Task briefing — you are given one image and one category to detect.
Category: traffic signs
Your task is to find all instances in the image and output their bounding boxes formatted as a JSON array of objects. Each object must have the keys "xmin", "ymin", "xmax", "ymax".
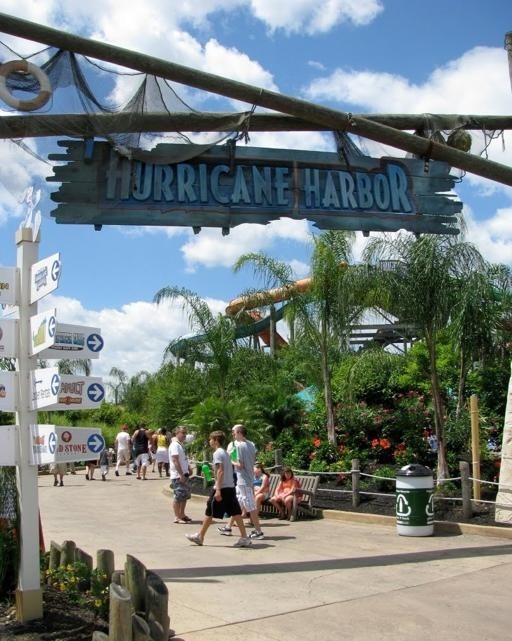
[
  {"xmin": 33, "ymin": 375, "xmax": 105, "ymax": 412},
  {"xmin": 29, "ymin": 308, "xmax": 58, "ymax": 357},
  {"xmin": 1, "ymin": 372, "xmax": 17, "ymax": 412},
  {"xmin": 1, "ymin": 320, "xmax": 18, "ymax": 357},
  {"xmin": 0, "ymin": 266, "xmax": 21, "ymax": 302},
  {"xmin": 30, "ymin": 423, "xmax": 56, "ymax": 464},
  {"xmin": 54, "ymin": 427, "xmax": 106, "ymax": 463},
  {"xmin": 27, "ymin": 367, "xmax": 59, "ymax": 415},
  {"xmin": 39, "ymin": 324, "xmax": 104, "ymax": 360},
  {"xmin": 30, "ymin": 254, "xmax": 63, "ymax": 304}
]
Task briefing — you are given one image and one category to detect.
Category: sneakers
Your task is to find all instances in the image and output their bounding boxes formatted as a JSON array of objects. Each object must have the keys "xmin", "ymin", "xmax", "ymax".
[
  {"xmin": 235, "ymin": 537, "xmax": 252, "ymax": 547},
  {"xmin": 185, "ymin": 533, "xmax": 203, "ymax": 545},
  {"xmin": 218, "ymin": 526, "xmax": 232, "ymax": 535},
  {"xmin": 249, "ymin": 531, "xmax": 265, "ymax": 539},
  {"xmin": 182, "ymin": 516, "xmax": 192, "ymax": 521}
]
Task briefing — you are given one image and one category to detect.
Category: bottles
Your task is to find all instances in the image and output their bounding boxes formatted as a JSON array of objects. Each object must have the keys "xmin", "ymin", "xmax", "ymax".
[
  {"xmin": 230, "ymin": 448, "xmax": 238, "ymax": 462},
  {"xmin": 201, "ymin": 463, "xmax": 212, "ymax": 483}
]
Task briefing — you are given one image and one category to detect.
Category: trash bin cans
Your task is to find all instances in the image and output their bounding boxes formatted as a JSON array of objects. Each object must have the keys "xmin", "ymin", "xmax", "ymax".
[{"xmin": 395, "ymin": 464, "xmax": 435, "ymax": 537}]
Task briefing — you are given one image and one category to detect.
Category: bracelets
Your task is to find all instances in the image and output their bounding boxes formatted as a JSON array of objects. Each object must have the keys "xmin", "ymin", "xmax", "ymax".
[{"xmin": 216, "ymin": 488, "xmax": 221, "ymax": 490}]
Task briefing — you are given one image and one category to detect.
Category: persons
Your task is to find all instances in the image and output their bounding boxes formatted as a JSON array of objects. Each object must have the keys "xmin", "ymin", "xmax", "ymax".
[
  {"xmin": 217, "ymin": 426, "xmax": 264, "ymax": 540},
  {"xmin": 269, "ymin": 468, "xmax": 304, "ymax": 520},
  {"xmin": 49, "ymin": 464, "xmax": 65, "ymax": 486},
  {"xmin": 184, "ymin": 431, "xmax": 252, "ymax": 547},
  {"xmin": 63, "ymin": 463, "xmax": 77, "ymax": 475},
  {"xmin": 201, "ymin": 424, "xmax": 257, "ymax": 527},
  {"xmin": 232, "ymin": 463, "xmax": 270, "ymax": 528},
  {"xmin": 85, "ymin": 422, "xmax": 195, "ymax": 524}
]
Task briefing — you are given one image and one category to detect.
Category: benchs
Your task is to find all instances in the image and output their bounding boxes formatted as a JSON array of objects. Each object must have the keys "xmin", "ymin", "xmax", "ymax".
[{"xmin": 260, "ymin": 473, "xmax": 323, "ymax": 522}]
